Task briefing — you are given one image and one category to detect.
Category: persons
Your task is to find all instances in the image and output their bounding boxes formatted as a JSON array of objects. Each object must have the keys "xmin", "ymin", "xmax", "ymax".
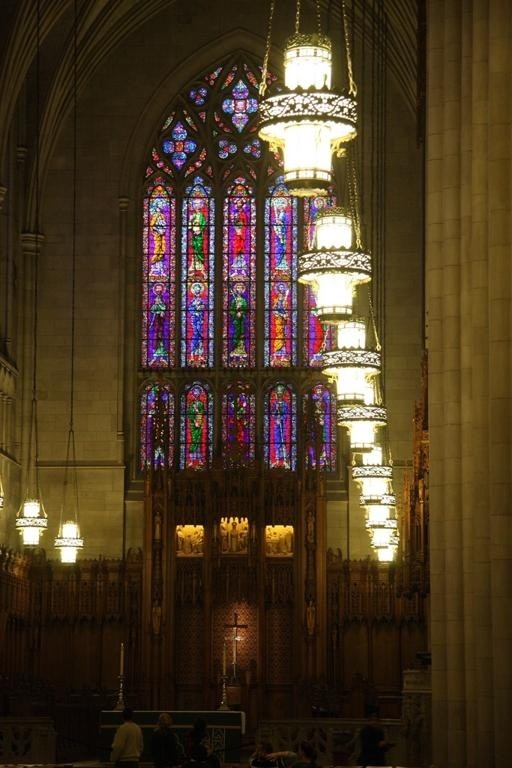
[{"xmin": 109, "ymin": 706, "xmax": 397, "ymax": 767}]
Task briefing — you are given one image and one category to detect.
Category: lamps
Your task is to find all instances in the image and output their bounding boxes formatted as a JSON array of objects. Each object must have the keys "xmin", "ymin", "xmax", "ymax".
[
  {"xmin": 258, "ymin": 1, "xmax": 360, "ymax": 202},
  {"xmin": 309, "ymin": 283, "xmax": 404, "ymax": 570},
  {"xmin": 297, "ymin": 140, "xmax": 372, "ymax": 319},
  {"xmin": 16, "ymin": 0, "xmax": 84, "ymax": 566}
]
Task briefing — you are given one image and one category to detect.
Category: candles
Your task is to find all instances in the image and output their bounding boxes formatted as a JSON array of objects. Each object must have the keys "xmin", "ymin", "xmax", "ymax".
[
  {"xmin": 222, "ymin": 643, "xmax": 227, "ymax": 675},
  {"xmin": 119, "ymin": 643, "xmax": 125, "ymax": 676}
]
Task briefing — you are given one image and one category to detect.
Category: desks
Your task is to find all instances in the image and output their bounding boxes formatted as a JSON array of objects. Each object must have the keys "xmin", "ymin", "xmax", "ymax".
[{"xmin": 99, "ymin": 710, "xmax": 247, "ymax": 760}]
